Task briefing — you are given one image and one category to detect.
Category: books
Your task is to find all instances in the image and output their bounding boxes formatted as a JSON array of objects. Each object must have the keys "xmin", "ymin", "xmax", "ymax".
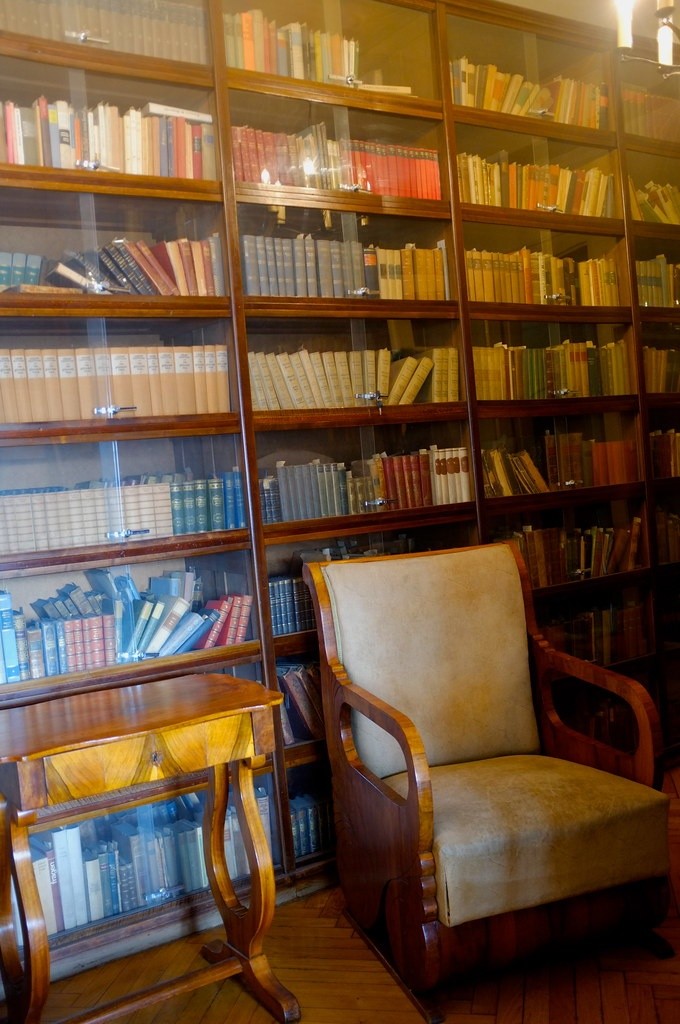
[{"xmin": 0, "ymin": 1, "xmax": 680, "ymax": 945}]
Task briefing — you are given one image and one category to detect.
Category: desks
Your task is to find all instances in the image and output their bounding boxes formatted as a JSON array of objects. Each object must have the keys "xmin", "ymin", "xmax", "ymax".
[{"xmin": 0, "ymin": 672, "xmax": 302, "ymax": 1024}]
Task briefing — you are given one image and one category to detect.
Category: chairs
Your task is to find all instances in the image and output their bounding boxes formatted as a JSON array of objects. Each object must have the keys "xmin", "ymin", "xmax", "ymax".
[{"xmin": 303, "ymin": 539, "xmax": 679, "ymax": 1024}]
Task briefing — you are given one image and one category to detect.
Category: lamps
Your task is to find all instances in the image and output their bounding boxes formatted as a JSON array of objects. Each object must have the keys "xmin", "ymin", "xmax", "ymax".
[{"xmin": 615, "ymin": 0, "xmax": 680, "ymax": 80}]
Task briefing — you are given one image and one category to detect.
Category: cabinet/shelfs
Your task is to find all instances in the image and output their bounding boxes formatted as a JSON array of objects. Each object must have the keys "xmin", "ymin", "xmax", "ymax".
[{"xmin": 0, "ymin": 2, "xmax": 680, "ymax": 1000}]
